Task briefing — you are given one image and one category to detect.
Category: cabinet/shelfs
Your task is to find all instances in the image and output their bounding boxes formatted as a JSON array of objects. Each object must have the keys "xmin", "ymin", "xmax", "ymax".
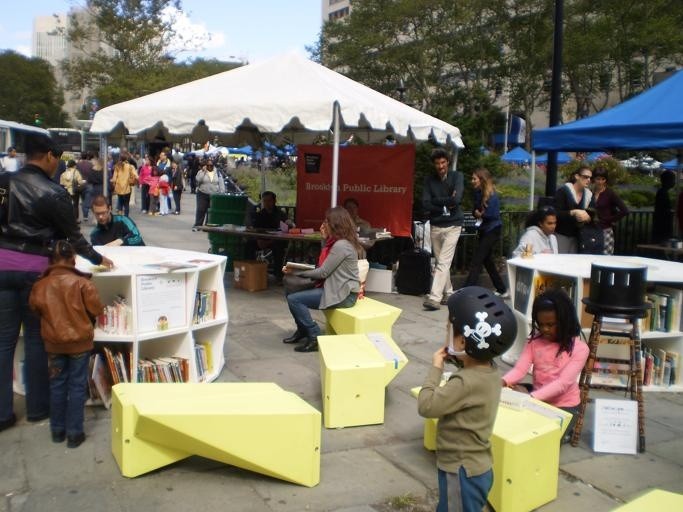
[
  {"xmin": 11, "ymin": 244, "xmax": 232, "ymax": 407},
  {"xmin": 498, "ymin": 253, "xmax": 682, "ymax": 394}
]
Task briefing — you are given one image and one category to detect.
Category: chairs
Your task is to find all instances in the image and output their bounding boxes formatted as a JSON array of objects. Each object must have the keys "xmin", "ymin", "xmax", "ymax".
[{"xmin": 568, "ymin": 261, "xmax": 654, "ymax": 454}]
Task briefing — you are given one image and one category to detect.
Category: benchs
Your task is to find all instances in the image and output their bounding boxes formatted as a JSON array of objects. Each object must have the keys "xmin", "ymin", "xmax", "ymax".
[
  {"xmin": 318, "ymin": 294, "xmax": 403, "ymax": 337},
  {"xmin": 610, "ymin": 486, "xmax": 682, "ymax": 512},
  {"xmin": 109, "ymin": 381, "xmax": 322, "ymax": 490},
  {"xmin": 408, "ymin": 373, "xmax": 574, "ymax": 512},
  {"xmin": 315, "ymin": 332, "xmax": 409, "ymax": 430}
]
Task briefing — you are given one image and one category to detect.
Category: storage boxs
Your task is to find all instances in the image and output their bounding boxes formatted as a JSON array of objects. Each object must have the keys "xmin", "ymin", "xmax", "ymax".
[
  {"xmin": 362, "ymin": 263, "xmax": 393, "ymax": 295},
  {"xmin": 233, "ymin": 258, "xmax": 268, "ymax": 293}
]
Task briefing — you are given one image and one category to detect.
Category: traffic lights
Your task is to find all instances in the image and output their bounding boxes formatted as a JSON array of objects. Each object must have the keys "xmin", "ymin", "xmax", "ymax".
[
  {"xmin": 34, "ymin": 113, "xmax": 39, "ymax": 123},
  {"xmin": 81, "ymin": 104, "xmax": 85, "ymax": 113}
]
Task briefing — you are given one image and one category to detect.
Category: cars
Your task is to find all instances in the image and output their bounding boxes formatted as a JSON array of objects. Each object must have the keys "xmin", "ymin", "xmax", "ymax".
[{"xmin": 180, "ymin": 160, "xmax": 189, "ymax": 178}]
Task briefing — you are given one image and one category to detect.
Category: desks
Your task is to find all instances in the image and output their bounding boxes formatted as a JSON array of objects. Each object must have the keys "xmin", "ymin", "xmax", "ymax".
[{"xmin": 189, "ymin": 223, "xmax": 393, "ymax": 284}]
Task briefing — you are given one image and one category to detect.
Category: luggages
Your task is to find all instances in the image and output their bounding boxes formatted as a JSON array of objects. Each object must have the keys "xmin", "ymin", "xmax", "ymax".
[{"xmin": 392, "ymin": 221, "xmax": 433, "ymax": 297}]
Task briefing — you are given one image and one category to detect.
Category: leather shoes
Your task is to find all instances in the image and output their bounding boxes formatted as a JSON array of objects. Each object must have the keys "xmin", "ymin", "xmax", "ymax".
[
  {"xmin": 283, "ymin": 331, "xmax": 305, "ymax": 344},
  {"xmin": 294, "ymin": 339, "xmax": 319, "ymax": 352}
]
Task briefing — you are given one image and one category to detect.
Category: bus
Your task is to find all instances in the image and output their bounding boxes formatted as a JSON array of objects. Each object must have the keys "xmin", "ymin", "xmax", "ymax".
[{"xmin": 0, "ymin": 119, "xmax": 173, "ymax": 175}]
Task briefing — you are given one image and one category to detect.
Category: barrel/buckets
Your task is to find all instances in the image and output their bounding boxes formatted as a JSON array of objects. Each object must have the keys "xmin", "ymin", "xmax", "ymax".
[{"xmin": 207, "ymin": 192, "xmax": 251, "ymax": 272}]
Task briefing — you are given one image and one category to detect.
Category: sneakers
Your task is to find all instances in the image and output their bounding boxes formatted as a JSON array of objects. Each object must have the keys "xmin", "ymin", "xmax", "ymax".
[
  {"xmin": 67, "ymin": 431, "xmax": 86, "ymax": 449},
  {"xmin": 26, "ymin": 412, "xmax": 51, "ymax": 423},
  {"xmin": 423, "ymin": 298, "xmax": 441, "ymax": 311},
  {"xmin": 440, "ymin": 294, "xmax": 451, "ymax": 305},
  {"xmin": 140, "ymin": 207, "xmax": 180, "ymax": 217},
  {"xmin": 275, "ymin": 278, "xmax": 283, "ymax": 287},
  {"xmin": 493, "ymin": 291, "xmax": 509, "ymax": 299},
  {"xmin": 0, "ymin": 412, "xmax": 17, "ymax": 433},
  {"xmin": 192, "ymin": 227, "xmax": 201, "ymax": 232},
  {"xmin": 50, "ymin": 428, "xmax": 67, "ymax": 444}
]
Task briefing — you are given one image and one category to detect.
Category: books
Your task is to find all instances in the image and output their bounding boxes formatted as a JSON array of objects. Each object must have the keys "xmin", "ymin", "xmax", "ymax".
[
  {"xmin": 284, "ymin": 262, "xmax": 316, "ymax": 271},
  {"xmin": 88, "ymin": 288, "xmax": 216, "ymax": 410},
  {"xmin": 594, "ymin": 285, "xmax": 683, "ymax": 386}
]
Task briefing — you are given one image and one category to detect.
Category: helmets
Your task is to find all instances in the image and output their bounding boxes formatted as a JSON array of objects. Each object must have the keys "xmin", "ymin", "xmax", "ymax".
[{"xmin": 446, "ymin": 284, "xmax": 519, "ymax": 361}]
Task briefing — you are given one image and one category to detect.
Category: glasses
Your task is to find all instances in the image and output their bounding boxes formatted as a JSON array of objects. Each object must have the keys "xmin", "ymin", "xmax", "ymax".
[
  {"xmin": 580, "ymin": 174, "xmax": 593, "ymax": 181},
  {"xmin": 541, "ymin": 204, "xmax": 554, "ymax": 211}
]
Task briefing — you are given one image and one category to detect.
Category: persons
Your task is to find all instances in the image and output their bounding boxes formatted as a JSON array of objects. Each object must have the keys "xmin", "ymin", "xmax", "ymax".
[
  {"xmin": 283, "ymin": 208, "xmax": 363, "ymax": 353},
  {"xmin": 462, "ymin": 168, "xmax": 512, "ymax": 300},
  {"xmin": 422, "ymin": 149, "xmax": 465, "ymax": 309},
  {"xmin": 0, "ymin": 134, "xmax": 114, "ymax": 431},
  {"xmin": 502, "ymin": 286, "xmax": 592, "ymax": 431},
  {"xmin": 651, "ymin": 170, "xmax": 675, "ymax": 244},
  {"xmin": 0, "ymin": 144, "xmax": 245, "ymax": 245},
  {"xmin": 417, "ymin": 286, "xmax": 517, "ymax": 512},
  {"xmin": 511, "ymin": 206, "xmax": 558, "ymax": 253},
  {"xmin": 245, "ymin": 190, "xmax": 288, "ymax": 268},
  {"xmin": 29, "ymin": 238, "xmax": 104, "ymax": 448},
  {"xmin": 343, "ymin": 196, "xmax": 370, "ymax": 230},
  {"xmin": 592, "ymin": 166, "xmax": 627, "ymax": 254},
  {"xmin": 555, "ymin": 167, "xmax": 595, "ymax": 252}
]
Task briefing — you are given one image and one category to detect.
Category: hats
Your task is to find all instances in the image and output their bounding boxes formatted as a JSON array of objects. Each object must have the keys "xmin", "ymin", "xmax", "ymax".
[{"xmin": 590, "ymin": 166, "xmax": 610, "ymax": 182}]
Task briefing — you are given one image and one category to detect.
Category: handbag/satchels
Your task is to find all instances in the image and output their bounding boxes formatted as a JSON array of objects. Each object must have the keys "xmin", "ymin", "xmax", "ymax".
[
  {"xmin": 575, "ymin": 215, "xmax": 605, "ymax": 255},
  {"xmin": 71, "ymin": 168, "xmax": 89, "ymax": 195},
  {"xmin": 128, "ymin": 164, "xmax": 136, "ymax": 187}
]
